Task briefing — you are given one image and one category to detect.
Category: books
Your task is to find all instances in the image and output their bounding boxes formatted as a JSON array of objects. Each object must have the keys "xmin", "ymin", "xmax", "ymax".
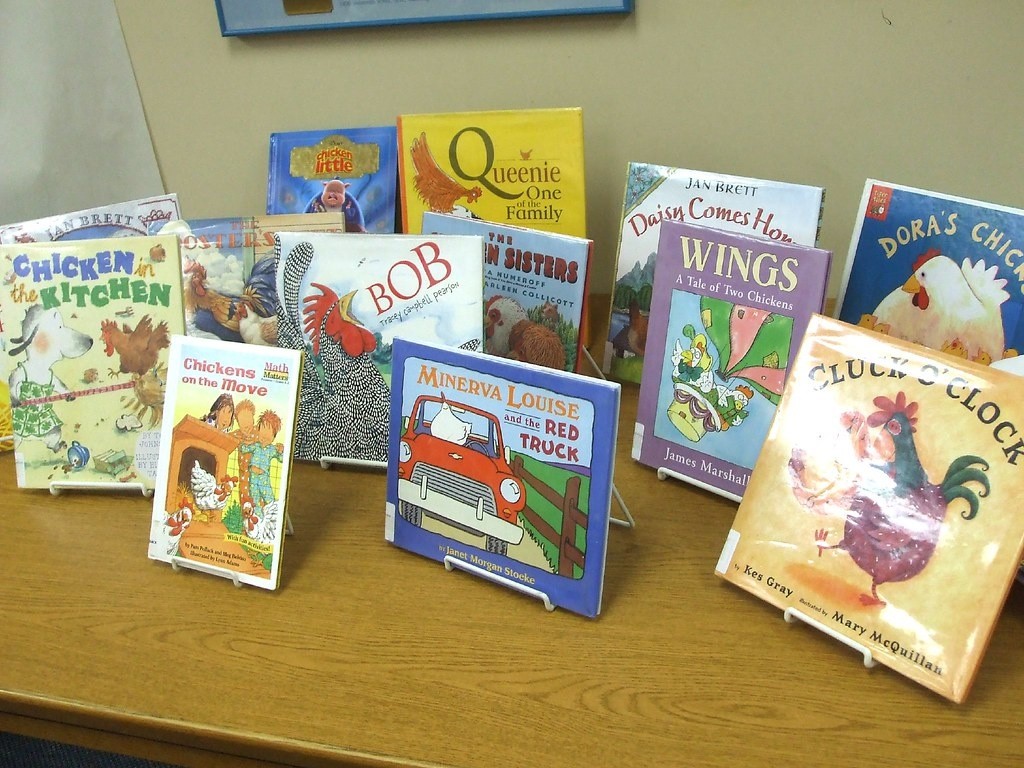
[{"xmin": 1, "ymin": 105, "xmax": 1024, "ymax": 706}]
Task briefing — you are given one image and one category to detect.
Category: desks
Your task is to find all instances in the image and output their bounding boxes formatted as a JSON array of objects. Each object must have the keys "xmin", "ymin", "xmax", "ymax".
[{"xmin": 0, "ymin": 290, "xmax": 1024, "ymax": 768}]
